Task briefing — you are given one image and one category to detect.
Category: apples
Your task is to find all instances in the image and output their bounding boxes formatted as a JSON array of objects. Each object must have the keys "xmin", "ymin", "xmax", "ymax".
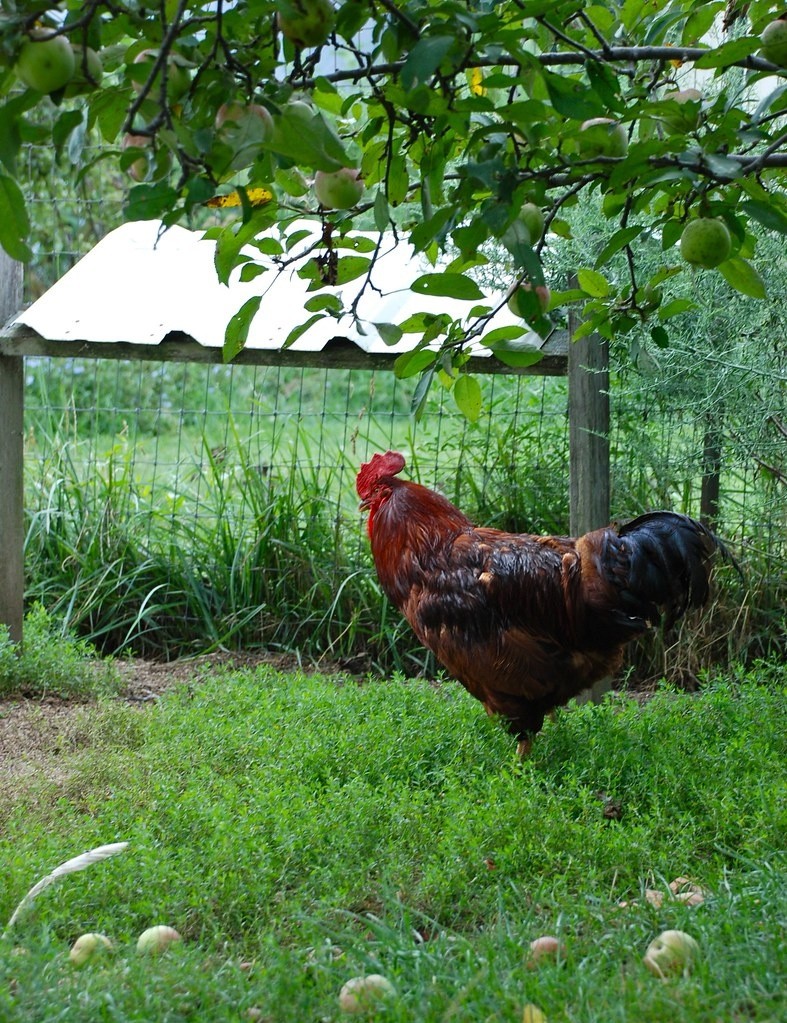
[
  {"xmin": 0, "ymin": 0, "xmax": 787, "ymax": 268},
  {"xmin": 8, "ymin": 875, "xmax": 714, "ymax": 1023}
]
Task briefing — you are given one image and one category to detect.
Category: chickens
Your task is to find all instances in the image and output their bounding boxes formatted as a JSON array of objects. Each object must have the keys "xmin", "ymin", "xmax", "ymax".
[{"xmin": 356, "ymin": 450, "xmax": 744, "ymax": 760}]
